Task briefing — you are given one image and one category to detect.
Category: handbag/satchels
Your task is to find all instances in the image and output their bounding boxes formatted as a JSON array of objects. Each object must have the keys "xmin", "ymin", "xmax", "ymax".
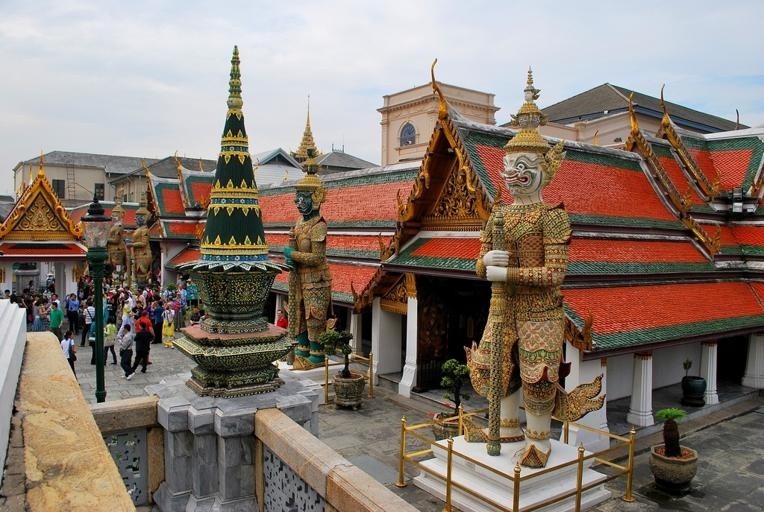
[{"xmin": 69, "ymin": 350, "xmax": 77, "ymax": 362}]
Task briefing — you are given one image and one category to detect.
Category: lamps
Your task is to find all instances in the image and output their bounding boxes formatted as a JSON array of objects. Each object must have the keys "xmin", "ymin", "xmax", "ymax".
[
  {"xmin": 730, "ymin": 185, "xmax": 745, "ymax": 201},
  {"xmin": 733, "ymin": 200, "xmax": 744, "ymax": 214}
]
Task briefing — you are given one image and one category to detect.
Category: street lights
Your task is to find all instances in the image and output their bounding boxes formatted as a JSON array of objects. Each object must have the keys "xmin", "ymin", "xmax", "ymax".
[
  {"xmin": 81, "ymin": 190, "xmax": 116, "ymax": 403},
  {"xmin": 115, "ymin": 263, "xmax": 123, "ymax": 282}
]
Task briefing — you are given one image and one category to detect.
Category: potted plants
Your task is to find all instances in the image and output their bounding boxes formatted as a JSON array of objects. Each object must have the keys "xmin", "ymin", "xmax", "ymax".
[
  {"xmin": 318, "ymin": 328, "xmax": 365, "ymax": 412},
  {"xmin": 679, "ymin": 356, "xmax": 707, "ymax": 407},
  {"xmin": 647, "ymin": 403, "xmax": 700, "ymax": 499},
  {"xmin": 431, "ymin": 355, "xmax": 470, "ymax": 440}
]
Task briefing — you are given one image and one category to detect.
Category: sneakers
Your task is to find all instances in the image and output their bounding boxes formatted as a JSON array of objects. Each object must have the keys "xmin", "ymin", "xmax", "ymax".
[{"xmin": 121, "ymin": 369, "xmax": 146, "ymax": 380}]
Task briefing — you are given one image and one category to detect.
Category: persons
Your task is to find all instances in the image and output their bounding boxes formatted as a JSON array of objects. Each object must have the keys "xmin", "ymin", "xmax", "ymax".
[
  {"xmin": 3, "ymin": 265, "xmax": 199, "ymax": 382},
  {"xmin": 285, "ymin": 148, "xmax": 333, "ymax": 373},
  {"xmin": 106, "ymin": 193, "xmax": 128, "ymax": 274},
  {"xmin": 276, "ymin": 310, "xmax": 288, "ymax": 363},
  {"xmin": 461, "ymin": 64, "xmax": 606, "ymax": 468},
  {"xmin": 125, "ymin": 190, "xmax": 154, "ymax": 281}
]
